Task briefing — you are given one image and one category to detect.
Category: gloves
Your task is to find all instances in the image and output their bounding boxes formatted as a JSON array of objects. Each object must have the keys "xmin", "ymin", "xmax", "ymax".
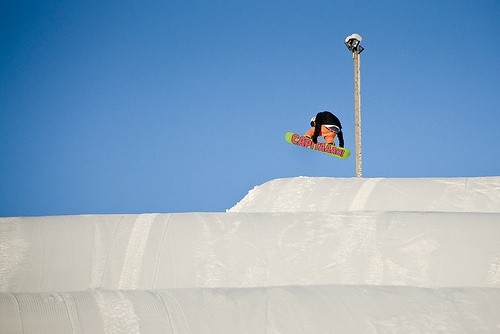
[
  {"xmin": 313, "ymin": 135, "xmax": 317, "ymax": 145},
  {"xmin": 339, "ymin": 141, "xmax": 345, "ymax": 147}
]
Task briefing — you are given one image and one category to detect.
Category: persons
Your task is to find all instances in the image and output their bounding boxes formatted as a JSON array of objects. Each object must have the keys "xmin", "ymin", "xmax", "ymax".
[{"xmin": 303, "ymin": 111, "xmax": 344, "ymax": 148}]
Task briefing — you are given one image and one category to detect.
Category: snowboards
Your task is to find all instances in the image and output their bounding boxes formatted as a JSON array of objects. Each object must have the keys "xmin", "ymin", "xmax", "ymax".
[{"xmin": 285, "ymin": 131, "xmax": 351, "ymax": 160}]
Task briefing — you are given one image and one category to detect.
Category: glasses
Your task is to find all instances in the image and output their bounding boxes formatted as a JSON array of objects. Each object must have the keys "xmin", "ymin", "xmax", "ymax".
[{"xmin": 310, "ymin": 121, "xmax": 314, "ymax": 126}]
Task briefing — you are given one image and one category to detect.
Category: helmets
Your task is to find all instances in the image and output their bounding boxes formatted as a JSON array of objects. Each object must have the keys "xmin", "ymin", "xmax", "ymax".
[{"xmin": 309, "ymin": 116, "xmax": 315, "ymax": 126}]
